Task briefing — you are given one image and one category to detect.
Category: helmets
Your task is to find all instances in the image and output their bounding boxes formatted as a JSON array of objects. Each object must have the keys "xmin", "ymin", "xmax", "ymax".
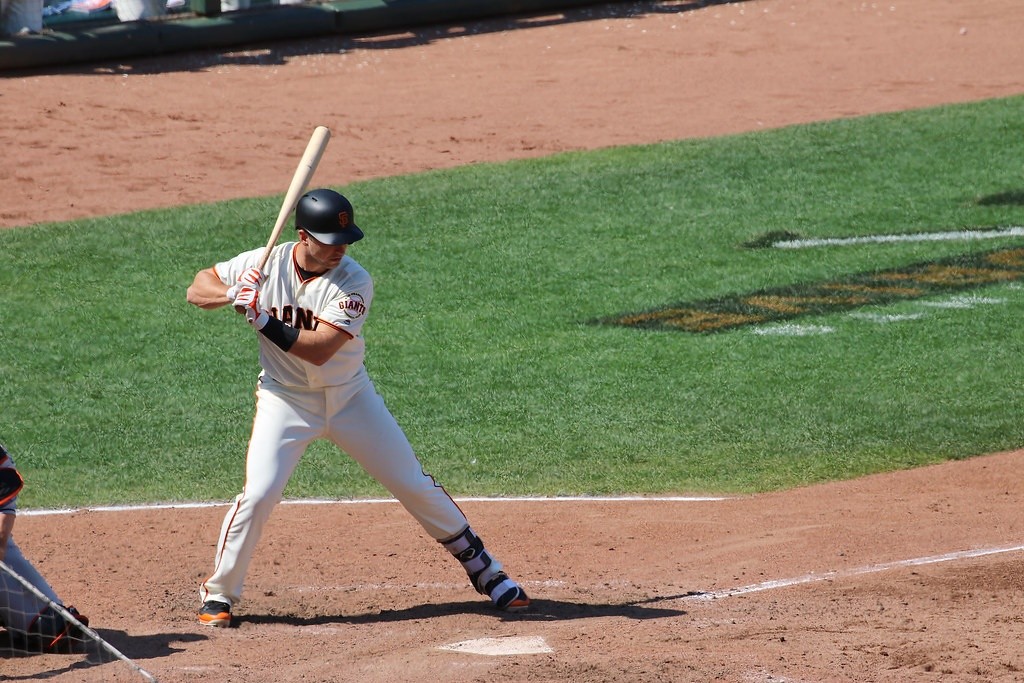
[{"xmin": 295, "ymin": 189, "xmax": 364, "ymax": 246}]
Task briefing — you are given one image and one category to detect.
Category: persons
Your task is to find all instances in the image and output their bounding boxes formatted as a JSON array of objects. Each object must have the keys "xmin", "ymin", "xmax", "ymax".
[
  {"xmin": 0, "ymin": 443, "xmax": 89, "ymax": 653},
  {"xmin": 186, "ymin": 190, "xmax": 531, "ymax": 628}
]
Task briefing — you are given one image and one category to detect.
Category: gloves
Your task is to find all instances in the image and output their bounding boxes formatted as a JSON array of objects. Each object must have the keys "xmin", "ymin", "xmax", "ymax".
[
  {"xmin": 226, "ymin": 268, "xmax": 271, "ymax": 303},
  {"xmin": 231, "ymin": 286, "xmax": 268, "ymax": 331}
]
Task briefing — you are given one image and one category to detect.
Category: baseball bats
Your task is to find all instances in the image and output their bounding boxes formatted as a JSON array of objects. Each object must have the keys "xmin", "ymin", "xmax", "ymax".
[{"xmin": 234, "ymin": 124, "xmax": 333, "ymax": 315}]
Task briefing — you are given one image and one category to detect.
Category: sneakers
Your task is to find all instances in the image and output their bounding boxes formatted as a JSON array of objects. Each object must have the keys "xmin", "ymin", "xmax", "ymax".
[
  {"xmin": 495, "ymin": 589, "xmax": 531, "ymax": 610},
  {"xmin": 197, "ymin": 600, "xmax": 232, "ymax": 629}
]
